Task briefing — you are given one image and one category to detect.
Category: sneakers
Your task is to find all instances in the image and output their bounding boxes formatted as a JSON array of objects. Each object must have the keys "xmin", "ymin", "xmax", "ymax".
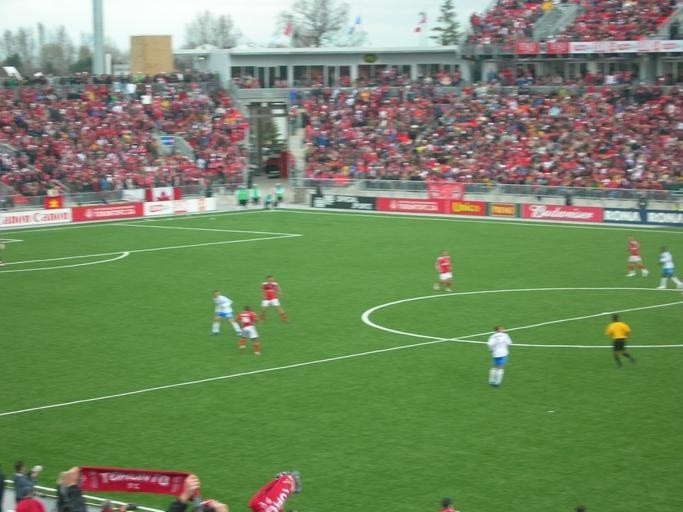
[{"xmin": 433, "ymin": 285, "xmax": 452, "ymax": 291}]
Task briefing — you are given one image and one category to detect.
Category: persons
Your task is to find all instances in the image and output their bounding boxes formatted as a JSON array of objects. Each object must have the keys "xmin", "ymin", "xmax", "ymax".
[
  {"xmin": 623, "ymin": 234, "xmax": 648, "ymax": 278},
  {"xmin": 235, "ymin": 307, "xmax": 261, "ymax": 355},
  {"xmin": 257, "ymin": 274, "xmax": 288, "ymax": 324},
  {"xmin": 487, "ymin": 326, "xmax": 515, "ymax": 388},
  {"xmin": 433, "ymin": 252, "xmax": 454, "ymax": 292},
  {"xmin": 209, "ymin": 290, "xmax": 241, "ymax": 335},
  {"xmin": 603, "ymin": 314, "xmax": 637, "ymax": 368},
  {"xmin": 439, "ymin": 497, "xmax": 460, "ymax": 512},
  {"xmin": 654, "ymin": 245, "xmax": 682, "ymax": 290}
]
